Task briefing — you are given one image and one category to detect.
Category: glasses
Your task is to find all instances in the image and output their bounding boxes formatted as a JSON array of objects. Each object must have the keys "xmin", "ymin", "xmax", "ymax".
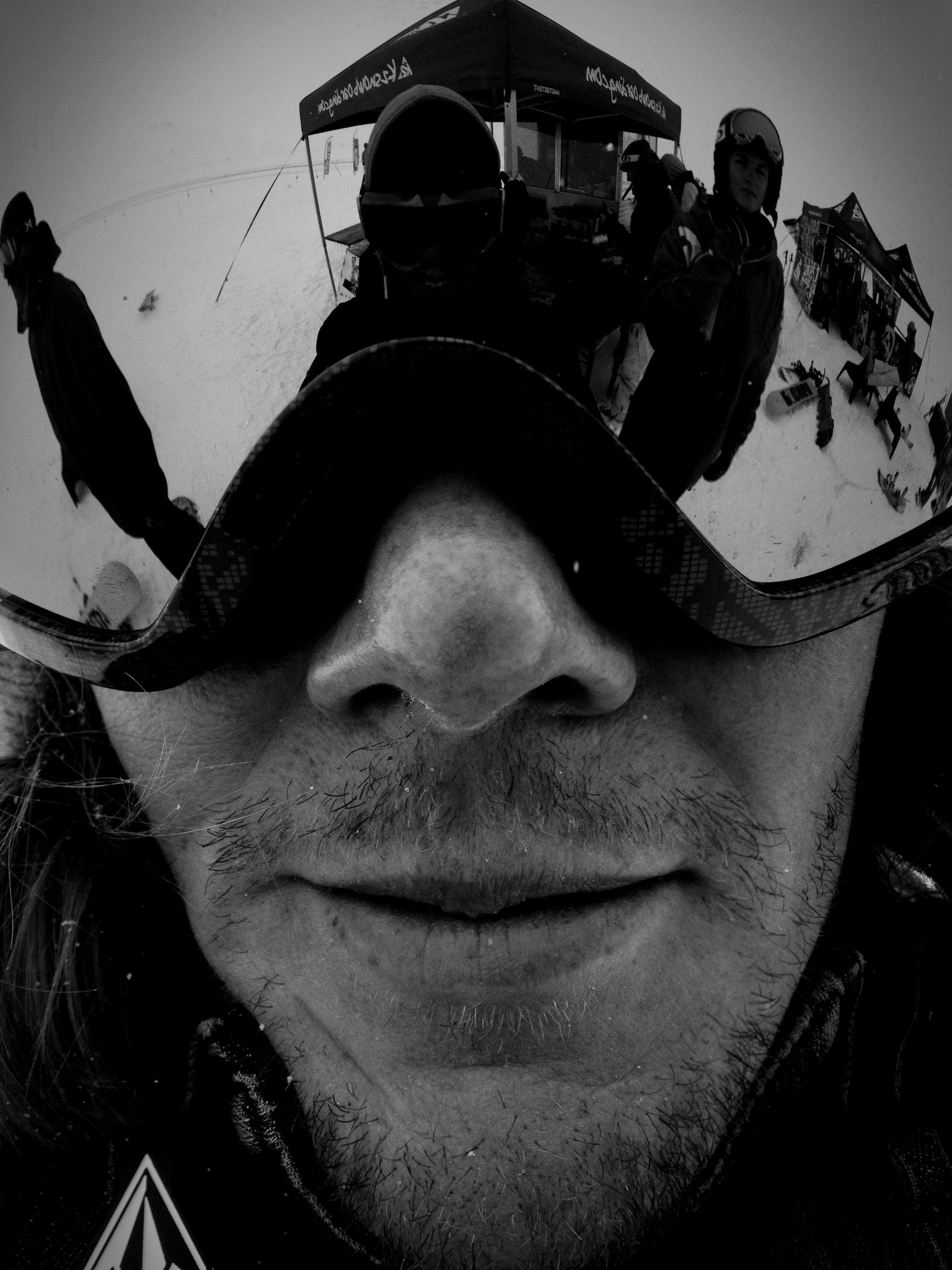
[{"xmin": 1, "ymin": 2, "xmax": 947, "ymax": 688}]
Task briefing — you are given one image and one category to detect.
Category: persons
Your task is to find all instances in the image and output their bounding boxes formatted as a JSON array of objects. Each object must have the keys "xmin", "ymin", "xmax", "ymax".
[
  {"xmin": 293, "ymin": 83, "xmax": 599, "ymax": 433},
  {"xmin": 0, "ymin": 0, "xmax": 952, "ymax": 1268},
  {"xmin": 2, "ymin": 189, "xmax": 208, "ymax": 581},
  {"xmin": 891, "ymin": 322, "xmax": 917, "ymax": 391},
  {"xmin": 600, "ymin": 139, "xmax": 708, "ymax": 424},
  {"xmin": 620, "ymin": 105, "xmax": 783, "ymax": 508}
]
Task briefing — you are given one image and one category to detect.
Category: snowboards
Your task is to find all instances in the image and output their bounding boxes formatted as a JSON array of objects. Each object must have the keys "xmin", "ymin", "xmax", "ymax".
[
  {"xmin": 766, "ymin": 377, "xmax": 818, "ymax": 417},
  {"xmin": 877, "ymin": 468, "xmax": 908, "ymax": 513},
  {"xmin": 79, "ymin": 560, "xmax": 143, "ymax": 629},
  {"xmin": 816, "ymin": 383, "xmax": 834, "ymax": 448}
]
